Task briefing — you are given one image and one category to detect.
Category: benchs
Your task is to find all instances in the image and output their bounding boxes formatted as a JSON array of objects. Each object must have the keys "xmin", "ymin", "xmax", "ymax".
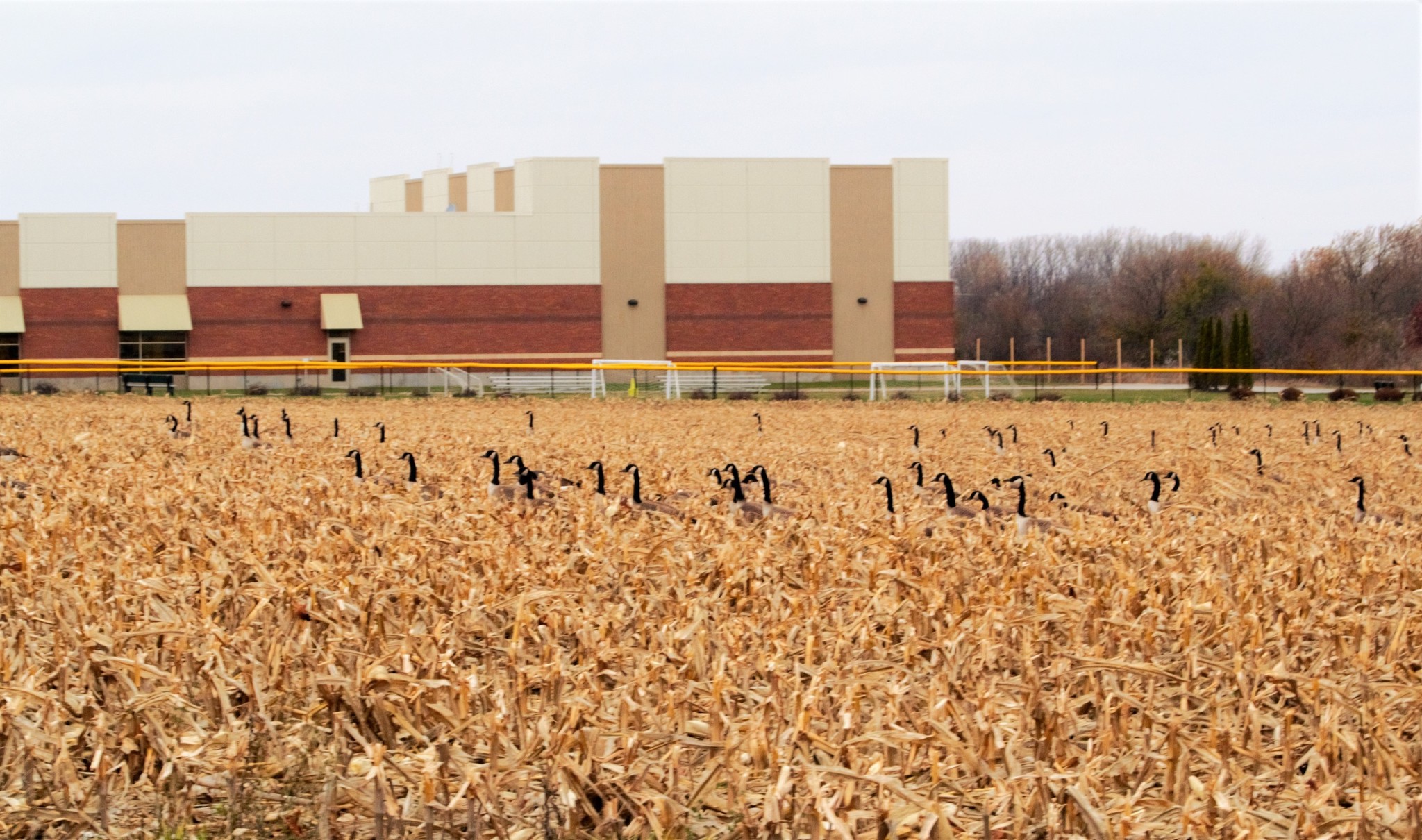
[
  {"xmin": 655, "ymin": 375, "xmax": 771, "ymax": 392},
  {"xmin": 121, "ymin": 373, "xmax": 175, "ymax": 396},
  {"xmin": 486, "ymin": 376, "xmax": 605, "ymax": 394}
]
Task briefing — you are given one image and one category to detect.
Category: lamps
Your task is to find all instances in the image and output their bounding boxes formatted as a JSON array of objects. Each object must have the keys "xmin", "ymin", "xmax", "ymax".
[
  {"xmin": 281, "ymin": 301, "xmax": 292, "ymax": 307},
  {"xmin": 629, "ymin": 298, "xmax": 639, "ymax": 306},
  {"xmin": 857, "ymin": 297, "xmax": 867, "ymax": 304}
]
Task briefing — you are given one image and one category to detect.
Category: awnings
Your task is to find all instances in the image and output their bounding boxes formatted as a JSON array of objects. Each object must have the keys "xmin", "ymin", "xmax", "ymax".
[
  {"xmin": 117, "ymin": 293, "xmax": 193, "ymax": 330},
  {"xmin": 0, "ymin": 296, "xmax": 26, "ymax": 332},
  {"xmin": 320, "ymin": 293, "xmax": 364, "ymax": 329}
]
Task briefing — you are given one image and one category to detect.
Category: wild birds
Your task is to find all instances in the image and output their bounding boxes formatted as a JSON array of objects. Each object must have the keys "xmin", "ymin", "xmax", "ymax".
[{"xmin": 163, "ymin": 398, "xmax": 1413, "ymax": 538}]
